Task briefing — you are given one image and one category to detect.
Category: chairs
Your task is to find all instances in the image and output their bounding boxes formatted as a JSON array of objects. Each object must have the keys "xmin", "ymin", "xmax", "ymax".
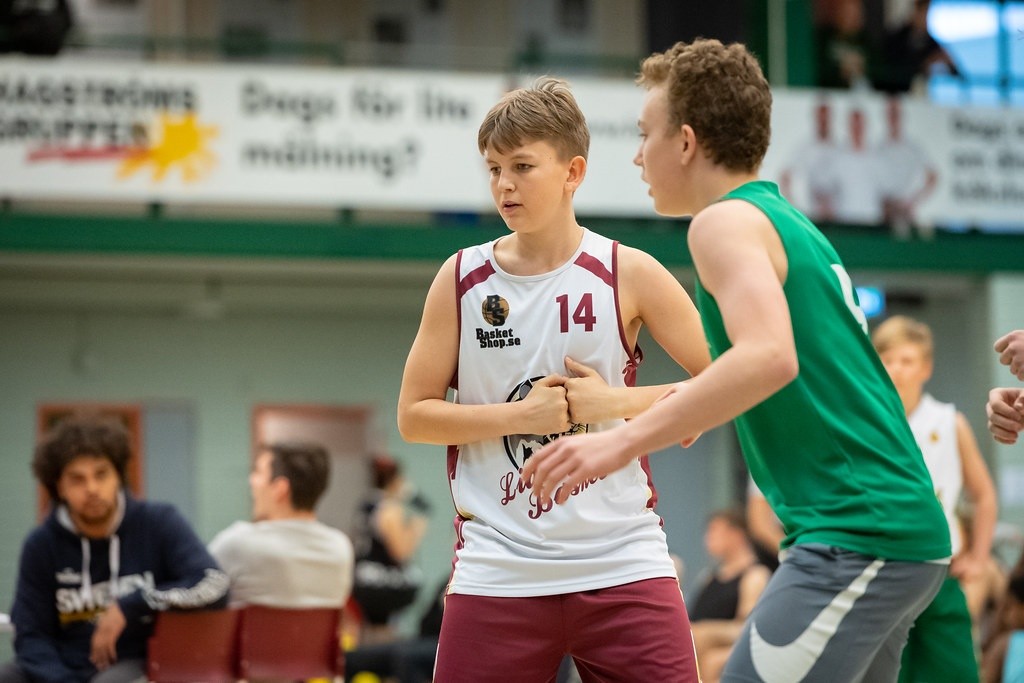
[{"xmin": 144, "ymin": 601, "xmax": 346, "ymax": 683}]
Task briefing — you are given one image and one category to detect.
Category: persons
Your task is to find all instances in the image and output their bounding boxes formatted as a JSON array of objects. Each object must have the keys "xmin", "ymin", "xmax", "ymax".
[
  {"xmin": 683, "ymin": 512, "xmax": 775, "ymax": 683},
  {"xmin": 742, "ymin": 308, "xmax": 1024, "ymax": 683},
  {"xmin": 397, "ymin": 74, "xmax": 717, "ymax": 683},
  {"xmin": 0, "ymin": 0, "xmax": 78, "ymax": 59},
  {"xmin": 520, "ymin": 37, "xmax": 955, "ymax": 683},
  {"xmin": 1, "ymin": 406, "xmax": 232, "ymax": 683},
  {"xmin": 807, "ymin": 0, "xmax": 965, "ymax": 98},
  {"xmin": 205, "ymin": 439, "xmax": 359, "ymax": 611},
  {"xmin": 352, "ymin": 452, "xmax": 430, "ymax": 647}
]
